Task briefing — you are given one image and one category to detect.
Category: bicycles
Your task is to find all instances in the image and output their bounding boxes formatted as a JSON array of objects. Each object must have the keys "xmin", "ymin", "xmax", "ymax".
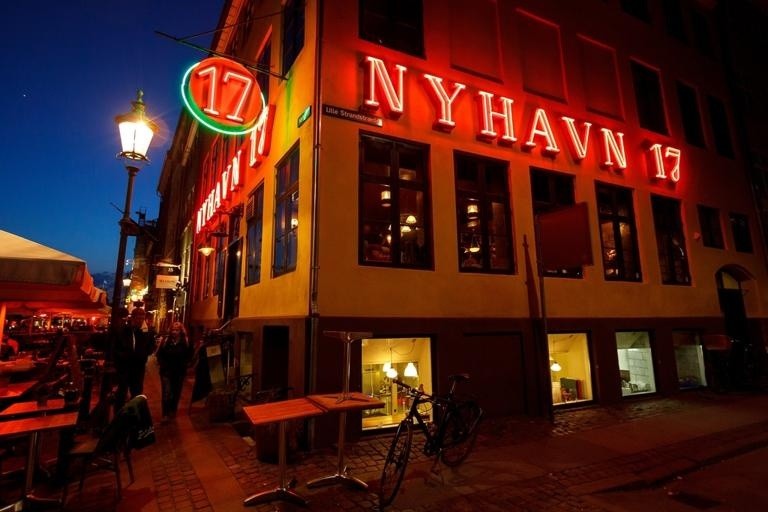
[{"xmin": 379, "ymin": 378, "xmax": 482, "ymax": 505}]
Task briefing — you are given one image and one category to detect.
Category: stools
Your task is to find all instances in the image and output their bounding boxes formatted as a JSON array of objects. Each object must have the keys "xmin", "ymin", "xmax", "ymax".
[{"xmin": 324, "ymin": 329, "xmax": 374, "ymax": 404}]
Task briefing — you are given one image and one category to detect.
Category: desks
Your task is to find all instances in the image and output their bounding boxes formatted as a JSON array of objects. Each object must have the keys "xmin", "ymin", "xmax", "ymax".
[
  {"xmin": 0, "ymin": 378, "xmax": 82, "ymax": 511},
  {"xmin": 243, "ymin": 390, "xmax": 385, "ymax": 507}
]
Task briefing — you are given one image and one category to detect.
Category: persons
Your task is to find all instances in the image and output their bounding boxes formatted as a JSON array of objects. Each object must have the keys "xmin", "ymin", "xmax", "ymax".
[
  {"xmin": 155, "ymin": 321, "xmax": 191, "ymax": 425},
  {"xmin": 112, "ymin": 307, "xmax": 157, "ymax": 411}
]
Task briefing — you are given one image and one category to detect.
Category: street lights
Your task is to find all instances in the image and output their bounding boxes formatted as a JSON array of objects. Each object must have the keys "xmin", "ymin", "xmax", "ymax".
[
  {"xmin": 99, "ymin": 91, "xmax": 161, "ymax": 311},
  {"xmin": 122, "ymin": 272, "xmax": 134, "ymax": 313}
]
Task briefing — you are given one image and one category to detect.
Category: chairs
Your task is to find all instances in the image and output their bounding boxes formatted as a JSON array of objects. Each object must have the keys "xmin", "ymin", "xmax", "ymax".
[{"xmin": 62, "ymin": 393, "xmax": 146, "ymax": 500}]
